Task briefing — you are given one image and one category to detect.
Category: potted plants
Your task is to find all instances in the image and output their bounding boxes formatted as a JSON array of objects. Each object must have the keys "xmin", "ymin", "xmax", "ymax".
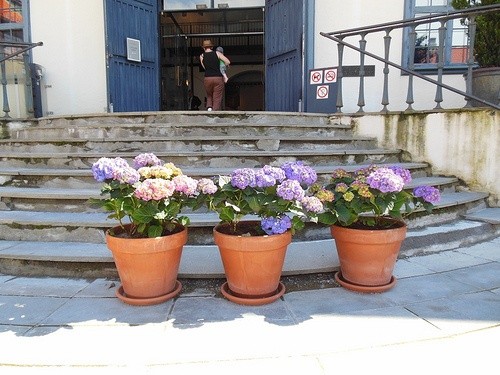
[{"xmin": 449, "ymin": 0, "xmax": 500, "ymax": 107}]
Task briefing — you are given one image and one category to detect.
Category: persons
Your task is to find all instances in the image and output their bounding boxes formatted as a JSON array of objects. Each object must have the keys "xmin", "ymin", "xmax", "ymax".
[
  {"xmin": 200, "ymin": 40, "xmax": 230, "ymax": 111},
  {"xmin": 187, "ymin": 90, "xmax": 201, "ymax": 111}
]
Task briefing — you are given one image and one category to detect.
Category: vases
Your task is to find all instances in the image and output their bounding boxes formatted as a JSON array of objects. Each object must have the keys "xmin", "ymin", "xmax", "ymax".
[
  {"xmin": 330, "ymin": 215, "xmax": 408, "ymax": 293},
  {"xmin": 212, "ymin": 221, "xmax": 292, "ymax": 305},
  {"xmin": 106, "ymin": 223, "xmax": 188, "ymax": 306}
]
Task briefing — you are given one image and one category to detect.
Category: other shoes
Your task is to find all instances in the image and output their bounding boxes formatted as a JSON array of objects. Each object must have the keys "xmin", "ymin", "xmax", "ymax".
[{"xmin": 207, "ymin": 107, "xmax": 212, "ymax": 112}]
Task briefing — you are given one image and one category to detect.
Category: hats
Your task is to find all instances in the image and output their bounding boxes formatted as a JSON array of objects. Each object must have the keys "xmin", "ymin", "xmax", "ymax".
[
  {"xmin": 216, "ymin": 47, "xmax": 223, "ymax": 53},
  {"xmin": 201, "ymin": 40, "xmax": 214, "ymax": 47}
]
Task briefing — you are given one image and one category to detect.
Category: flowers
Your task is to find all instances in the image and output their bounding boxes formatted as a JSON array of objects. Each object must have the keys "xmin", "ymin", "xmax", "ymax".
[
  {"xmin": 316, "ymin": 163, "xmax": 441, "ymax": 230},
  {"xmin": 196, "ymin": 160, "xmax": 324, "ymax": 236},
  {"xmin": 87, "ymin": 151, "xmax": 200, "ymax": 238}
]
